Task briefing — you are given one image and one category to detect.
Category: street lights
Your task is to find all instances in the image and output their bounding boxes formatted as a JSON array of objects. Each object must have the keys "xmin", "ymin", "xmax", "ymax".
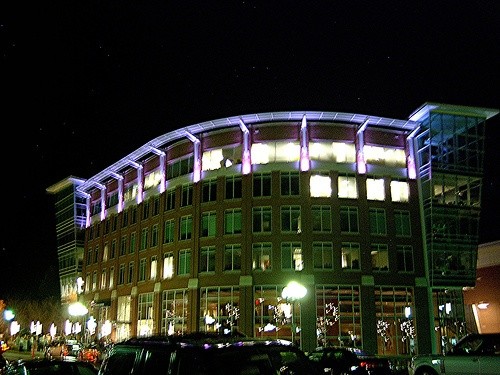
[{"xmin": 281, "ymin": 278, "xmax": 308, "ymax": 348}]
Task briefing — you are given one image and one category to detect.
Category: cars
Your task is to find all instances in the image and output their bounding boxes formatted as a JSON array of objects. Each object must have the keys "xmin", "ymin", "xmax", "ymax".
[
  {"xmin": 304, "ymin": 346, "xmax": 390, "ymax": 375},
  {"xmin": 407, "ymin": 333, "xmax": 499, "ymax": 374},
  {"xmin": 0, "ymin": 332, "xmax": 313, "ymax": 375}
]
